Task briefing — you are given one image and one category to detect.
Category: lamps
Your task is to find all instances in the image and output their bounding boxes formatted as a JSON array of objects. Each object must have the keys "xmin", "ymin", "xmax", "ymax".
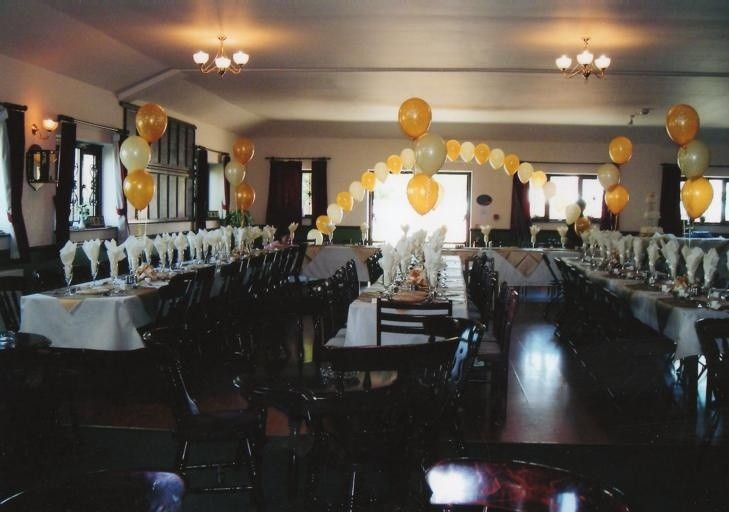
[
  {"xmin": 26, "ymin": 143, "xmax": 59, "ymax": 192},
  {"xmin": 30, "ymin": 117, "xmax": 59, "ymax": 141},
  {"xmin": 554, "ymin": 37, "xmax": 612, "ymax": 82},
  {"xmin": 191, "ymin": 31, "xmax": 251, "ymax": 79}
]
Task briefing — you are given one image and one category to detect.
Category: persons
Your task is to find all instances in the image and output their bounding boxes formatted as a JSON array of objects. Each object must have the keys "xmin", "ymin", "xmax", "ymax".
[{"xmin": 263, "ymin": 225, "xmax": 291, "ymax": 251}]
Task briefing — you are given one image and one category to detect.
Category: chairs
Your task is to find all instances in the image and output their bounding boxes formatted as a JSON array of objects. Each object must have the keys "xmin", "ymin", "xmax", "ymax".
[{"xmin": 1, "ymin": 223, "xmax": 728, "ymax": 511}]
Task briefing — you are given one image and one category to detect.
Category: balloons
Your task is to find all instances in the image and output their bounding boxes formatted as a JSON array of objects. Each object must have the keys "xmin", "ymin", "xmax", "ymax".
[
  {"xmin": 446, "ymin": 141, "xmax": 558, "ymax": 200},
  {"xmin": 565, "ymin": 203, "xmax": 581, "ymax": 225},
  {"xmin": 119, "ymin": 101, "xmax": 168, "ymax": 212},
  {"xmin": 408, "ymin": 175, "xmax": 438, "ymax": 215},
  {"xmin": 307, "ymin": 146, "xmax": 415, "ymax": 245},
  {"xmin": 597, "ymin": 135, "xmax": 634, "ymax": 215},
  {"xmin": 665, "ymin": 103, "xmax": 713, "ymax": 220},
  {"xmin": 398, "ymin": 98, "xmax": 432, "ymax": 140},
  {"xmin": 575, "ymin": 217, "xmax": 588, "ymax": 234},
  {"xmin": 414, "ymin": 133, "xmax": 446, "ymax": 178},
  {"xmin": 224, "ymin": 137, "xmax": 256, "ymax": 210}
]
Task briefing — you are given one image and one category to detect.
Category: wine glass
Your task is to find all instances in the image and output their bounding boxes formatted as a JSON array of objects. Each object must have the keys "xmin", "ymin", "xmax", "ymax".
[
  {"xmin": 378, "ymin": 247, "xmax": 450, "ymax": 302},
  {"xmin": 63, "ymin": 235, "xmax": 274, "ymax": 296},
  {"xmin": 531, "ymin": 234, "xmax": 536, "ymax": 249},
  {"xmin": 580, "ymin": 240, "xmax": 728, "ymax": 311},
  {"xmin": 561, "ymin": 236, "xmax": 567, "ymax": 248},
  {"xmin": 483, "ymin": 234, "xmax": 489, "ymax": 249}
]
